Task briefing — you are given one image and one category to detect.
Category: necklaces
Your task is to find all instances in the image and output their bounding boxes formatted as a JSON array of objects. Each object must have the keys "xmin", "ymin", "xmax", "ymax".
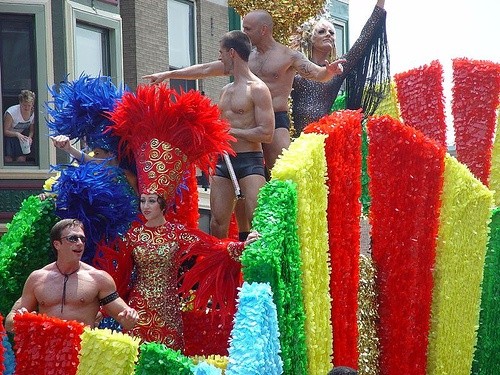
[{"xmin": 53, "ymin": 260, "xmax": 81, "ymax": 314}]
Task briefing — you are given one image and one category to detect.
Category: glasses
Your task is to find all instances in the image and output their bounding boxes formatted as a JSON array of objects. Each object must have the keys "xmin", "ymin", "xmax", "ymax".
[{"xmin": 57, "ymin": 235, "xmax": 86, "ymax": 243}]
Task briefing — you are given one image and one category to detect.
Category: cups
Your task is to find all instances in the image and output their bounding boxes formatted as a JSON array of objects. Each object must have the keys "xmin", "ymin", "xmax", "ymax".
[{"xmin": 18, "ymin": 137, "xmax": 31, "ymax": 154}]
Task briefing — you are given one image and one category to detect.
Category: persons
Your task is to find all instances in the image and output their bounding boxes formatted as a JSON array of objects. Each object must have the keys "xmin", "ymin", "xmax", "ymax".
[
  {"xmin": 4, "ymin": 89, "xmax": 37, "ymax": 165},
  {"xmin": 208, "ymin": 30, "xmax": 276, "ymax": 240},
  {"xmin": 291, "ymin": 0, "xmax": 389, "ymax": 136},
  {"xmin": 4, "ymin": 218, "xmax": 139, "ymax": 336},
  {"xmin": 94, "ymin": 180, "xmax": 259, "ymax": 356},
  {"xmin": 140, "ymin": 8, "xmax": 348, "ymax": 242},
  {"xmin": 51, "ymin": 120, "xmax": 141, "ymax": 202}
]
